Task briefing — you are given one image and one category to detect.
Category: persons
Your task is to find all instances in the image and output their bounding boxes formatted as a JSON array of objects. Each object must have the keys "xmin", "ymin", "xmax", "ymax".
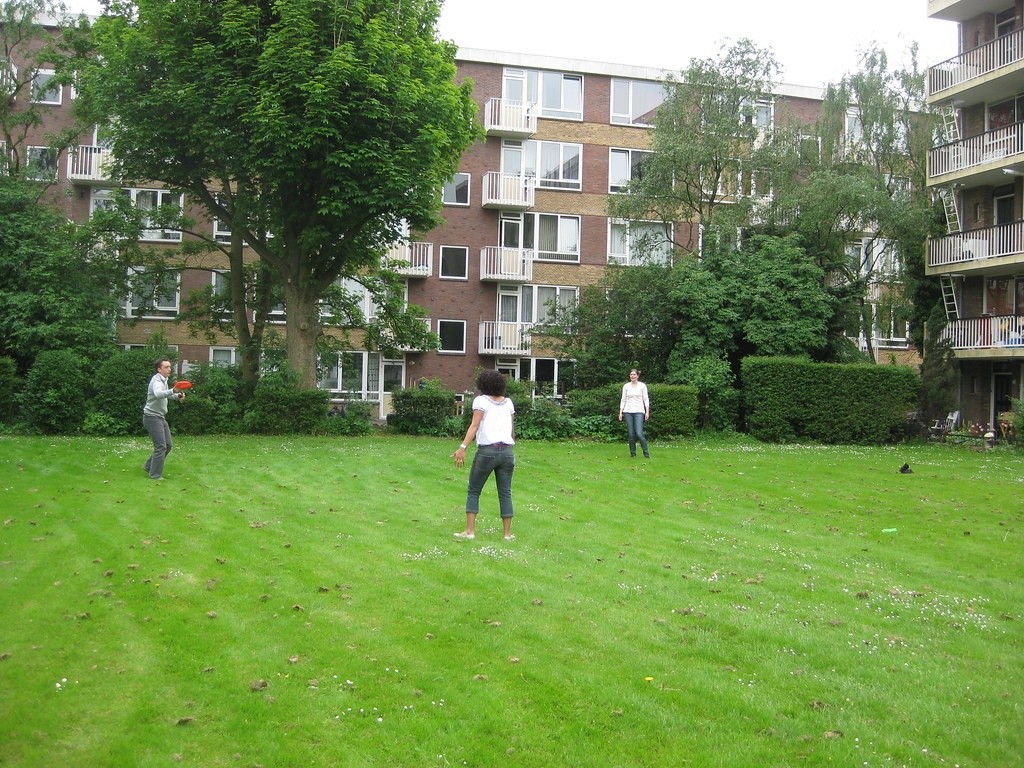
[
  {"xmin": 142, "ymin": 358, "xmax": 185, "ymax": 480},
  {"xmin": 449, "ymin": 369, "xmax": 516, "ymax": 540},
  {"xmin": 618, "ymin": 368, "xmax": 649, "ymax": 458}
]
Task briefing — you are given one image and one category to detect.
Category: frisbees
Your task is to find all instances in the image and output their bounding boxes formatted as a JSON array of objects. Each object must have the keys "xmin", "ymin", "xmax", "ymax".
[{"xmin": 175, "ymin": 381, "xmax": 191, "ymax": 389}]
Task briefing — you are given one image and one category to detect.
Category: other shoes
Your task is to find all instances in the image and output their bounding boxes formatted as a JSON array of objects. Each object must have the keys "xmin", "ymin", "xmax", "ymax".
[
  {"xmin": 454, "ymin": 531, "xmax": 475, "ymax": 539},
  {"xmin": 505, "ymin": 534, "xmax": 516, "ymax": 540},
  {"xmin": 149, "ymin": 474, "xmax": 164, "ymax": 480}
]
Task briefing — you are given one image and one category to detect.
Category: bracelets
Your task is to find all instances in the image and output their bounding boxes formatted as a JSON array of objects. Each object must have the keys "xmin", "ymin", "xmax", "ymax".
[{"xmin": 460, "ymin": 444, "xmax": 467, "ymax": 449}]
